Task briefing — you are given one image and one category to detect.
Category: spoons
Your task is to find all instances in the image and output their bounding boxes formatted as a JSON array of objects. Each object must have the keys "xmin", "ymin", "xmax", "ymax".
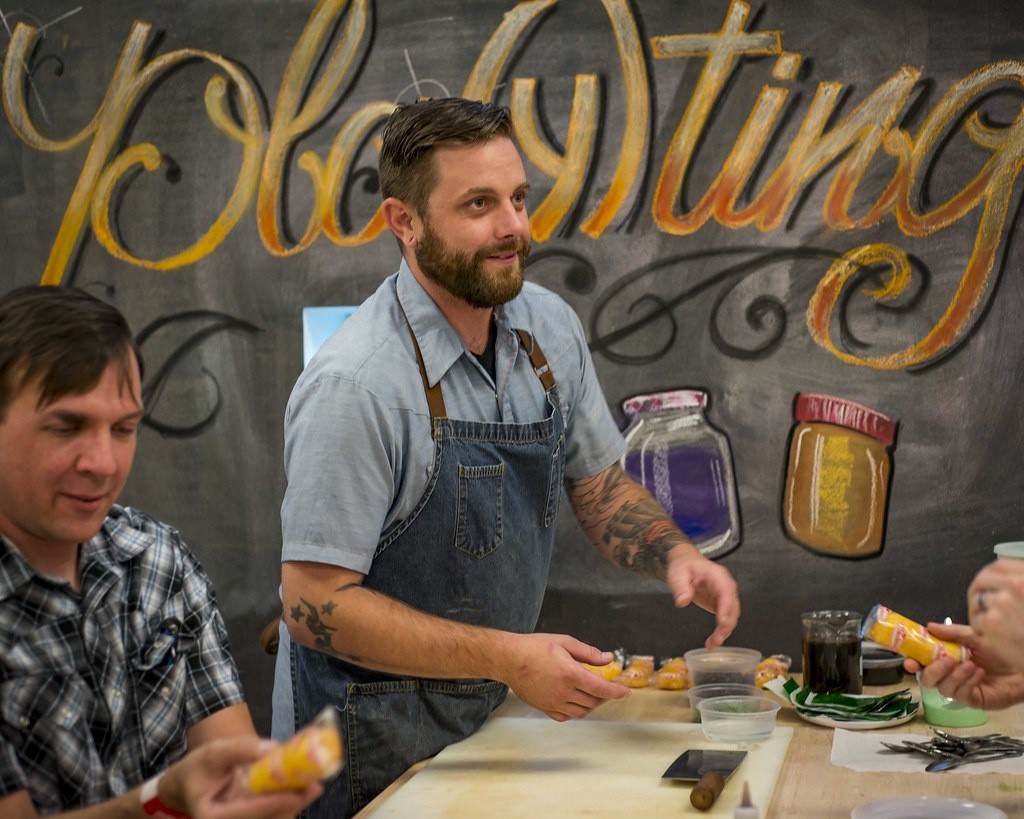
[{"xmin": 879, "ymin": 727, "xmax": 1024, "ymax": 774}]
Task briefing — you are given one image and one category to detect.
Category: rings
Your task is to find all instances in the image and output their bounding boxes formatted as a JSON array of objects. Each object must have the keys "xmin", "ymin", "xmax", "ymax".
[{"xmin": 978, "ymin": 587, "xmax": 987, "ymax": 614}]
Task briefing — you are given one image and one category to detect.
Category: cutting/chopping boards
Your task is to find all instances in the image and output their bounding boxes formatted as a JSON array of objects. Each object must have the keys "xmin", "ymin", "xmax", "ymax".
[{"xmin": 366, "ymin": 715, "xmax": 795, "ymax": 818}]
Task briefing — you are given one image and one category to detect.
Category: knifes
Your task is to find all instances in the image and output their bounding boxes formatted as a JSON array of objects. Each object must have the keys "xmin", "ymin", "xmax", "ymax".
[{"xmin": 661, "ymin": 750, "xmax": 751, "ymax": 811}]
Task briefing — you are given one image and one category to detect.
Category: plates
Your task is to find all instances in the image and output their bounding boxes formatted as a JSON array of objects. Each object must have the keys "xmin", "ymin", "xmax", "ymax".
[{"xmin": 851, "ymin": 796, "xmax": 1009, "ymax": 819}]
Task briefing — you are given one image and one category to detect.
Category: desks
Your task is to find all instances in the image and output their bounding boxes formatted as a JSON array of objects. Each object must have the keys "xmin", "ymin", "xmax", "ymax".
[{"xmin": 351, "ymin": 660, "xmax": 1024, "ymax": 819}]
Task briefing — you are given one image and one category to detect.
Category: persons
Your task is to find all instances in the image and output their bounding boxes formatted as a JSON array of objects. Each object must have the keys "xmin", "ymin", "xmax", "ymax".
[
  {"xmin": 280, "ymin": 99, "xmax": 747, "ymax": 790},
  {"xmin": 903, "ymin": 555, "xmax": 1024, "ymax": 708},
  {"xmin": 0, "ymin": 279, "xmax": 324, "ymax": 819}
]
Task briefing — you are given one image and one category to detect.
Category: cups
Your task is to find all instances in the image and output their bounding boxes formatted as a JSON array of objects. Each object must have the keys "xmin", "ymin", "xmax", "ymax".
[{"xmin": 802, "ymin": 608, "xmax": 864, "ymax": 694}]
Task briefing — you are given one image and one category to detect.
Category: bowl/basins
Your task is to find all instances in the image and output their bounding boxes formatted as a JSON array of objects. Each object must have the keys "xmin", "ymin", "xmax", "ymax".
[
  {"xmin": 684, "ymin": 646, "xmax": 763, "ymax": 693},
  {"xmin": 685, "ymin": 682, "xmax": 765, "ymax": 724},
  {"xmin": 698, "ymin": 695, "xmax": 780, "ymax": 742}
]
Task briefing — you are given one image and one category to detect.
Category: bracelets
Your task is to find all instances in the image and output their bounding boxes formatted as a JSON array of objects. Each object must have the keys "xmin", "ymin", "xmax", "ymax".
[{"xmin": 141, "ymin": 773, "xmax": 190, "ymax": 819}]
